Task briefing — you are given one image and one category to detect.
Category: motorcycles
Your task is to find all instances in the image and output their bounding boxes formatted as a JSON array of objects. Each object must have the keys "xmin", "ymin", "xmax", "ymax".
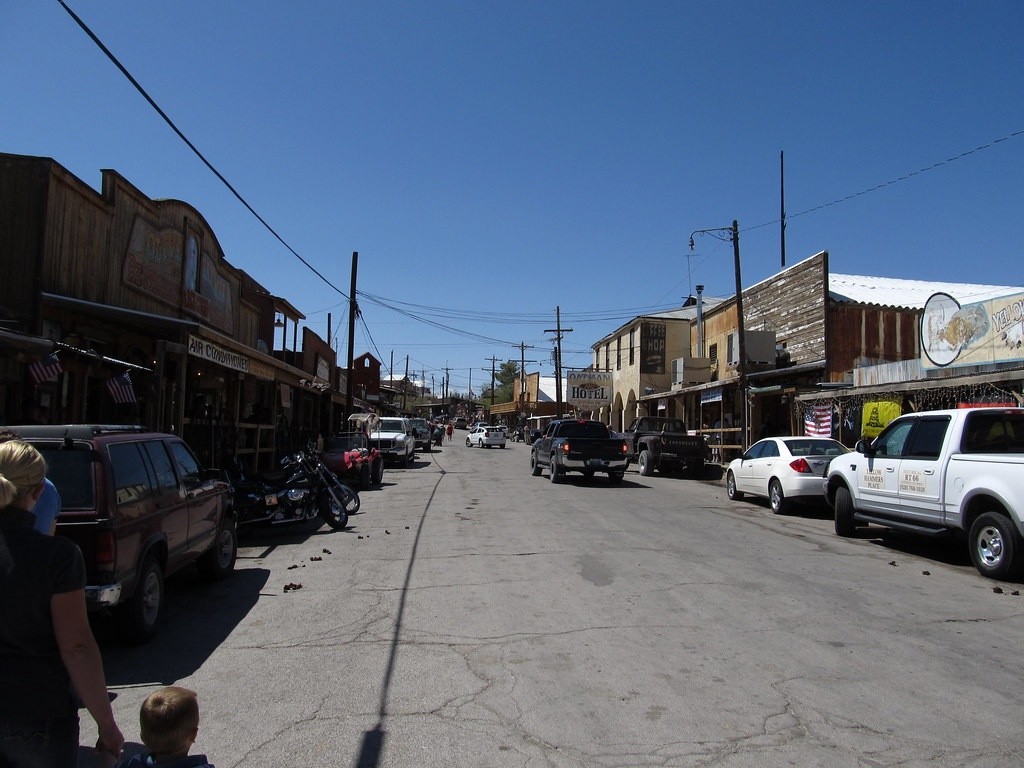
[{"xmin": 219, "ymin": 437, "xmax": 359, "ymax": 537}]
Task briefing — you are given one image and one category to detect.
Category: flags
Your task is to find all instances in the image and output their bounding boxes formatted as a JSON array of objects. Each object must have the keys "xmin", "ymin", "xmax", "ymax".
[
  {"xmin": 26, "ymin": 353, "xmax": 65, "ymax": 386},
  {"xmin": 804, "ymin": 405, "xmax": 832, "ymax": 437},
  {"xmin": 105, "ymin": 372, "xmax": 136, "ymax": 403}
]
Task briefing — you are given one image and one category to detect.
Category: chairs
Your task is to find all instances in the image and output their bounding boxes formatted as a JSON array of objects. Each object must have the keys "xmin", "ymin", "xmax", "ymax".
[
  {"xmin": 825, "ymin": 449, "xmax": 841, "ymax": 455},
  {"xmin": 791, "ymin": 448, "xmax": 807, "ymax": 456}
]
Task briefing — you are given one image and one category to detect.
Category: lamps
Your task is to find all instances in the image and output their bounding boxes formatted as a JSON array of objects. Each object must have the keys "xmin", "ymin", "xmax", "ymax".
[{"xmin": 274, "ymin": 313, "xmax": 285, "ymax": 327}]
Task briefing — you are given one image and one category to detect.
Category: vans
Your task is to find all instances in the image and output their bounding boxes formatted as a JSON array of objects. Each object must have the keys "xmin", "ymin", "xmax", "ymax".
[{"xmin": 451, "ymin": 416, "xmax": 467, "ymax": 430}]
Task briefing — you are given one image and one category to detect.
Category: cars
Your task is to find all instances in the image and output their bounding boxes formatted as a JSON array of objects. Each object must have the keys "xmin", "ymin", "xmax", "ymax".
[
  {"xmin": 497, "ymin": 425, "xmax": 508, "ymax": 434},
  {"xmin": 466, "ymin": 425, "xmax": 506, "ymax": 449},
  {"xmin": 725, "ymin": 436, "xmax": 852, "ymax": 514},
  {"xmin": 435, "ymin": 415, "xmax": 449, "ymax": 424},
  {"xmin": 471, "ymin": 422, "xmax": 491, "ymax": 432},
  {"xmin": 526, "ymin": 428, "xmax": 544, "ymax": 445}
]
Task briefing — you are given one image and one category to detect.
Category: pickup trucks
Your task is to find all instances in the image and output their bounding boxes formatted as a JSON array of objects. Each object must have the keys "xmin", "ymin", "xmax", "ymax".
[
  {"xmin": 618, "ymin": 416, "xmax": 706, "ymax": 478},
  {"xmin": 822, "ymin": 405, "xmax": 1024, "ymax": 580},
  {"xmin": 530, "ymin": 419, "xmax": 627, "ymax": 485}
]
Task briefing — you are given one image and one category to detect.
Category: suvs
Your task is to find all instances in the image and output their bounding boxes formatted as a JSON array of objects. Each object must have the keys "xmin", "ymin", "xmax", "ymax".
[
  {"xmin": 0, "ymin": 423, "xmax": 238, "ymax": 648},
  {"xmin": 354, "ymin": 416, "xmax": 417, "ymax": 469},
  {"xmin": 322, "ymin": 431, "xmax": 384, "ymax": 490},
  {"xmin": 408, "ymin": 417, "xmax": 432, "ymax": 452}
]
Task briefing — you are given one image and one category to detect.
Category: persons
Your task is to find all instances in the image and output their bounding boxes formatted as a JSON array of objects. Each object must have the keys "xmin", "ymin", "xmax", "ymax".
[
  {"xmin": 446, "ymin": 423, "xmax": 454, "ymax": 441},
  {"xmin": 0, "ymin": 439, "xmax": 124, "ymax": 768},
  {"xmin": 439, "ymin": 423, "xmax": 446, "ymax": 441},
  {"xmin": 115, "ymin": 686, "xmax": 215, "ymax": 768},
  {"xmin": 694, "ymin": 417, "xmax": 748, "ymax": 463}
]
